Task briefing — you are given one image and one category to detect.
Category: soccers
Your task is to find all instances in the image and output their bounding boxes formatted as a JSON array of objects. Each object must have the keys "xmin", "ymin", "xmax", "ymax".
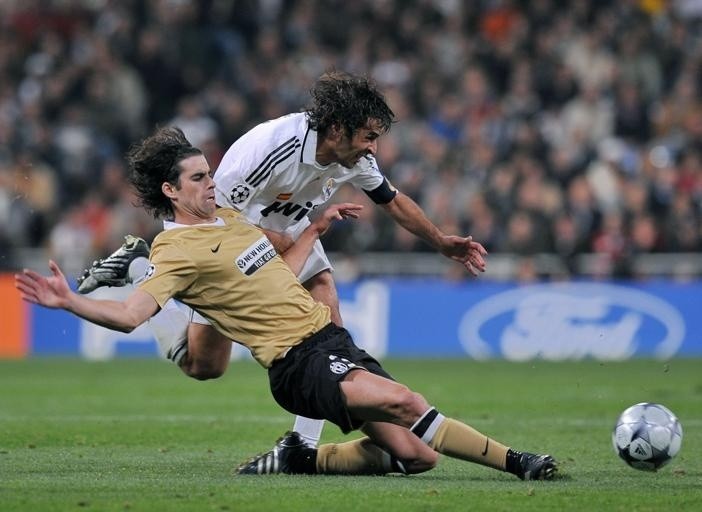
[{"xmin": 613, "ymin": 401, "xmax": 683, "ymax": 471}]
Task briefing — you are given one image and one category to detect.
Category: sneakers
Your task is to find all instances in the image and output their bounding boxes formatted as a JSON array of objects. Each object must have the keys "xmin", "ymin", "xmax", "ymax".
[
  {"xmin": 234, "ymin": 431, "xmax": 308, "ymax": 476},
  {"xmin": 519, "ymin": 451, "xmax": 558, "ymax": 480},
  {"xmin": 77, "ymin": 234, "xmax": 153, "ymax": 294}
]
